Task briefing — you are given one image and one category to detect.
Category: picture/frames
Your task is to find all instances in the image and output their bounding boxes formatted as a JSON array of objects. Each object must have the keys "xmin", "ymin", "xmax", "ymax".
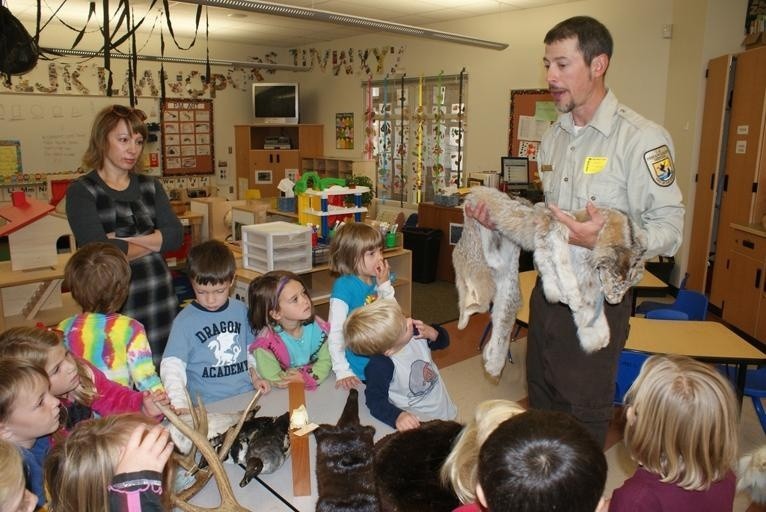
[
  {"xmin": 509, "ymin": 88, "xmax": 561, "ymax": 194},
  {"xmin": 160, "ymin": 97, "xmax": 215, "ymax": 177}
]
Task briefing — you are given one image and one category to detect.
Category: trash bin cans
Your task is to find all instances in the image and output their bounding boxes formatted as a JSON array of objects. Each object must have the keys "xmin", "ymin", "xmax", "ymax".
[{"xmin": 401, "ymin": 226, "xmax": 443, "ymax": 284}]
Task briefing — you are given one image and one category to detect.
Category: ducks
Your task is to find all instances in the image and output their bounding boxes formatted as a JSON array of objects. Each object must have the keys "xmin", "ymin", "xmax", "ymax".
[{"xmin": 193, "ymin": 406, "xmax": 292, "ymax": 487}]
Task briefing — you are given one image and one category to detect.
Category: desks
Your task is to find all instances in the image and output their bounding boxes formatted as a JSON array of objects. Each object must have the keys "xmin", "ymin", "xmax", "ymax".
[{"xmin": 0, "ymin": 197, "xmax": 766, "ymax": 512}]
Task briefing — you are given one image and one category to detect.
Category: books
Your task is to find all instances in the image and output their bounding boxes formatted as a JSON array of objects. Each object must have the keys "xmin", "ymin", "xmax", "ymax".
[{"xmin": 470, "ymin": 172, "xmax": 500, "ymax": 189}]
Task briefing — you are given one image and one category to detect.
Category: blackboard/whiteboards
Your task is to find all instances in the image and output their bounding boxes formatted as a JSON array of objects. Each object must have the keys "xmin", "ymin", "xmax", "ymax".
[{"xmin": 0, "ymin": 92, "xmax": 163, "ymax": 185}]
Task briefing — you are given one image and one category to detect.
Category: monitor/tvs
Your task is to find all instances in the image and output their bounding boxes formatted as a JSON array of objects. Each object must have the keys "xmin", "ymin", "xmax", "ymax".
[
  {"xmin": 251, "ymin": 81, "xmax": 300, "ymax": 125},
  {"xmin": 500, "ymin": 156, "xmax": 530, "ymax": 186}
]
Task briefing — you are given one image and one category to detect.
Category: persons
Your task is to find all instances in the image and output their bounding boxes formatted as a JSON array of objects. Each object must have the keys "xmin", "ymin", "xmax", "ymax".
[
  {"xmin": 342, "ymin": 299, "xmax": 459, "ymax": 432},
  {"xmin": 1, "ymin": 240, "xmax": 177, "ymax": 512},
  {"xmin": 159, "ymin": 222, "xmax": 399, "ymax": 416},
  {"xmin": 525, "ymin": 17, "xmax": 686, "ymax": 451},
  {"xmin": 440, "ymin": 352, "xmax": 766, "ymax": 512},
  {"xmin": 66, "ymin": 105, "xmax": 185, "ymax": 375}
]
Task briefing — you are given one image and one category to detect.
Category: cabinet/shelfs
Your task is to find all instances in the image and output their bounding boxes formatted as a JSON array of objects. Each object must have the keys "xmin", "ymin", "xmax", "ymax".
[
  {"xmin": 686, "ymin": 46, "xmax": 766, "ymax": 316},
  {"xmin": 235, "ymin": 125, "xmax": 325, "ymax": 200},
  {"xmin": 299, "ymin": 154, "xmax": 377, "ymax": 197}
]
